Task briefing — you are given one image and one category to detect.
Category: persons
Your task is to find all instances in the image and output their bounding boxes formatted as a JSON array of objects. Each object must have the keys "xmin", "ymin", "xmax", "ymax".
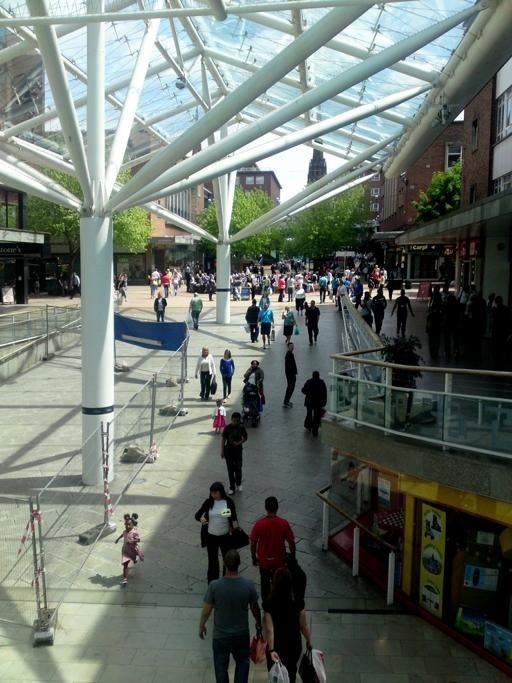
[
  {"xmin": 147, "ymin": 262, "xmax": 217, "ymax": 301},
  {"xmin": 154, "ymin": 292, "xmax": 167, "ymax": 323},
  {"xmin": 221, "ymin": 412, "xmax": 248, "ymax": 494},
  {"xmin": 250, "ymin": 496, "xmax": 296, "ymax": 601},
  {"xmin": 118, "ymin": 272, "xmax": 128, "ymax": 301},
  {"xmin": 230, "ymin": 259, "xmax": 511, "ymax": 371},
  {"xmin": 263, "ymin": 569, "xmax": 313, "ymax": 682},
  {"xmin": 198, "ymin": 549, "xmax": 262, "ymax": 683},
  {"xmin": 115, "ymin": 513, "xmax": 144, "ymax": 583},
  {"xmin": 30, "ymin": 270, "xmax": 81, "ymax": 299},
  {"xmin": 189, "ymin": 293, "xmax": 203, "ymax": 330},
  {"xmin": 195, "ymin": 481, "xmax": 240, "ymax": 584},
  {"xmin": 195, "ymin": 342, "xmax": 327, "ymax": 437}
]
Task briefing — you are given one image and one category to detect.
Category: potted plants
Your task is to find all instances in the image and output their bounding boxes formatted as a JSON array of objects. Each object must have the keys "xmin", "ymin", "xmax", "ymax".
[{"xmin": 381, "ymin": 333, "xmax": 426, "ymax": 387}]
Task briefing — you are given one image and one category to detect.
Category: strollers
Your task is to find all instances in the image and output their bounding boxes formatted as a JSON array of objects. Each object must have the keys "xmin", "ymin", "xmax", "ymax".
[{"xmin": 242, "ymin": 381, "xmax": 262, "ymax": 427}]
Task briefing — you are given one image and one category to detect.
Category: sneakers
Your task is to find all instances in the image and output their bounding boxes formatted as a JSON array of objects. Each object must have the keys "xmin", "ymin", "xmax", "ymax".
[
  {"xmin": 228, "ymin": 490, "xmax": 235, "ymax": 495},
  {"xmin": 238, "ymin": 485, "xmax": 243, "ymax": 492}
]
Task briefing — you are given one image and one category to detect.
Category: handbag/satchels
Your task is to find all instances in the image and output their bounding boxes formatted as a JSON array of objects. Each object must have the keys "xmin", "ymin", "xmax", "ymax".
[
  {"xmin": 210, "ymin": 376, "xmax": 217, "ymax": 394},
  {"xmin": 250, "ymin": 630, "xmax": 267, "ymax": 664},
  {"xmin": 230, "ymin": 526, "xmax": 250, "ymax": 551},
  {"xmin": 298, "ymin": 648, "xmax": 319, "ymax": 683},
  {"xmin": 271, "ymin": 330, "xmax": 275, "ymax": 341}
]
